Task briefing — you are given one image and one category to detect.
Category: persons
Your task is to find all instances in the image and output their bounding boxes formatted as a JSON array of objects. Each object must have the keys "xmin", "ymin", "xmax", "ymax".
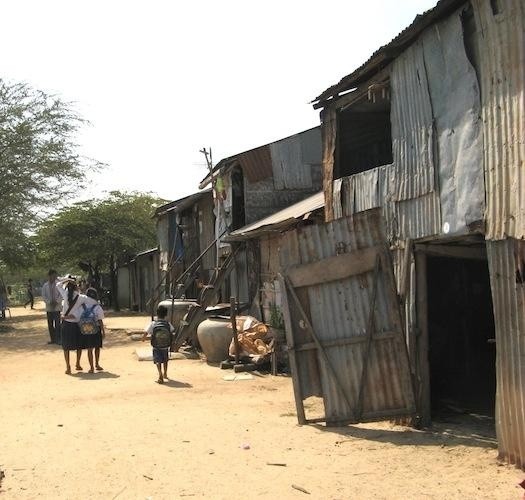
[
  {"xmin": 56, "ymin": 286, "xmax": 105, "ymax": 374},
  {"xmin": 41, "ymin": 269, "xmax": 64, "ymax": 345},
  {"xmin": 55, "ymin": 278, "xmax": 100, "ymax": 375},
  {"xmin": 22, "ymin": 278, "xmax": 35, "ymax": 309},
  {"xmin": 141, "ymin": 305, "xmax": 175, "ymax": 383}
]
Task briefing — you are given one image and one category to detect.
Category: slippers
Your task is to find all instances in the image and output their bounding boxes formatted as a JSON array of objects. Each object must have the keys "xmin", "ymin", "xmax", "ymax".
[
  {"xmin": 66, "ymin": 365, "xmax": 103, "ymax": 374},
  {"xmin": 157, "ymin": 377, "xmax": 164, "ymax": 384}
]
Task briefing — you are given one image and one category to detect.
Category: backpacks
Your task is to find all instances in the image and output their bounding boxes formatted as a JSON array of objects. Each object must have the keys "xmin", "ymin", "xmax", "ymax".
[
  {"xmin": 81, "ymin": 304, "xmax": 99, "ymax": 336},
  {"xmin": 151, "ymin": 321, "xmax": 171, "ymax": 348}
]
